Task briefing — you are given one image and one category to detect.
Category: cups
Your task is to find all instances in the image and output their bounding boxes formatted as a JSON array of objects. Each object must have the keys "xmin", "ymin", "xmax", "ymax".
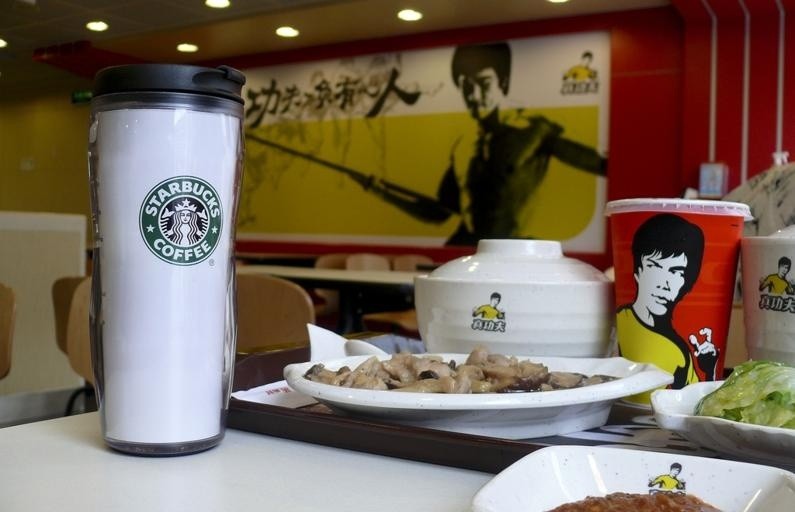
[
  {"xmin": 604, "ymin": 198, "xmax": 752, "ymax": 407},
  {"xmin": 70, "ymin": 63, "xmax": 247, "ymax": 455}
]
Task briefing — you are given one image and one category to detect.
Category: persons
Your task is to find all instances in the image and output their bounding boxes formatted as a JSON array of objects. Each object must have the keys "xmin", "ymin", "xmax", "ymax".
[
  {"xmin": 353, "ymin": 42, "xmax": 608, "ymax": 246},
  {"xmin": 619, "ymin": 214, "xmax": 720, "ymax": 406},
  {"xmin": 649, "ymin": 462, "xmax": 686, "ymax": 491},
  {"xmin": 563, "ymin": 51, "xmax": 596, "ymax": 81},
  {"xmin": 168, "ymin": 200, "xmax": 204, "ymax": 245},
  {"xmin": 472, "ymin": 293, "xmax": 506, "ymax": 321},
  {"xmin": 761, "ymin": 256, "xmax": 793, "ymax": 295}
]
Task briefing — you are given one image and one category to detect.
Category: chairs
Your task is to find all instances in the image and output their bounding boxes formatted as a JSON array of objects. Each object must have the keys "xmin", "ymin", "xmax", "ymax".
[
  {"xmin": 346, "ymin": 253, "xmax": 390, "ymax": 272},
  {"xmin": 0, "ymin": 283, "xmax": 15, "ymax": 379},
  {"xmin": 51, "ymin": 277, "xmax": 98, "ymax": 417},
  {"xmin": 393, "ymin": 255, "xmax": 434, "ymax": 271},
  {"xmin": 315, "ymin": 254, "xmax": 347, "ymax": 269},
  {"xmin": 233, "ymin": 271, "xmax": 315, "ymax": 357}
]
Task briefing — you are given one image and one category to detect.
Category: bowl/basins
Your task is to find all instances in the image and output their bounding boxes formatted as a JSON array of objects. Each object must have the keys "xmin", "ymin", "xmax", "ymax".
[
  {"xmin": 414, "ymin": 239, "xmax": 614, "ymax": 357},
  {"xmin": 740, "ymin": 221, "xmax": 795, "ymax": 366}
]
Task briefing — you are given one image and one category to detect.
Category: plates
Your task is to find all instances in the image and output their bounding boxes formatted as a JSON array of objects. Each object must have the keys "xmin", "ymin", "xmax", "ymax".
[
  {"xmin": 470, "ymin": 446, "xmax": 795, "ymax": 512},
  {"xmin": 650, "ymin": 380, "xmax": 795, "ymax": 464},
  {"xmin": 283, "ymin": 351, "xmax": 675, "ymax": 439}
]
